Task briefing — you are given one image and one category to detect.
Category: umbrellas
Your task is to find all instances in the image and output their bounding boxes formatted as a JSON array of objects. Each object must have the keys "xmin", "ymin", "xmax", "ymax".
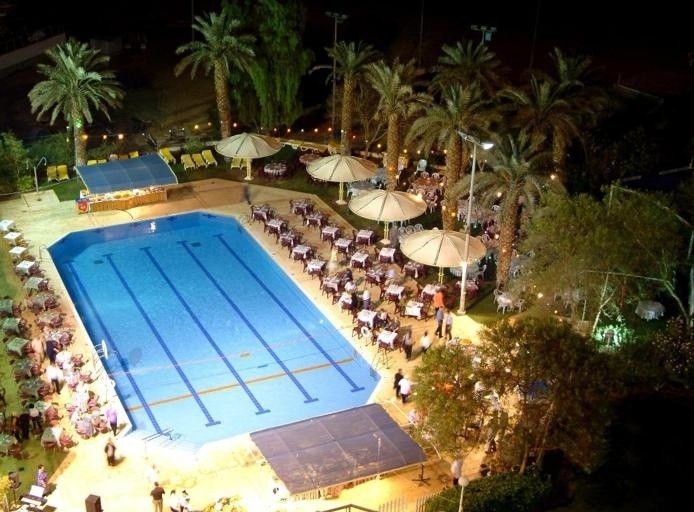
[
  {"xmin": 400, "ymin": 228, "xmax": 487, "ymax": 283},
  {"xmin": 306, "ymin": 152, "xmax": 380, "ymax": 201},
  {"xmin": 214, "ymin": 132, "xmax": 282, "ymax": 179},
  {"xmin": 345, "ymin": 188, "xmax": 430, "ymax": 240}
]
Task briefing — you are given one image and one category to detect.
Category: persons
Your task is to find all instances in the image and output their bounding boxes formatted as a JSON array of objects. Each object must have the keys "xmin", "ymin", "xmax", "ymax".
[
  {"xmin": 45, "ymin": 363, "xmax": 61, "ymax": 397},
  {"xmin": 484, "ymin": 409, "xmax": 498, "ymax": 455},
  {"xmin": 179, "ymin": 490, "xmax": 191, "ymax": 512},
  {"xmin": 27, "ymin": 401, "xmax": 44, "ymax": 434},
  {"xmin": 45, "ymin": 336, "xmax": 58, "ymax": 367},
  {"xmin": 450, "ymin": 452, "xmax": 462, "ymax": 486},
  {"xmin": 149, "ymin": 481, "xmax": 165, "ymax": 512},
  {"xmin": 9, "ymin": 412, "xmax": 24, "ymax": 443},
  {"xmin": 168, "ymin": 488, "xmax": 181, "ymax": 512},
  {"xmin": 102, "ymin": 403, "xmax": 119, "ymax": 436},
  {"xmin": 35, "ymin": 461, "xmax": 50, "ymax": 498},
  {"xmin": 342, "ymin": 263, "xmax": 454, "ymax": 406},
  {"xmin": 104, "ymin": 436, "xmax": 115, "ymax": 467},
  {"xmin": 32, "ymin": 334, "xmax": 42, "ymax": 366},
  {"xmin": 496, "ymin": 407, "xmax": 510, "ymax": 444}
]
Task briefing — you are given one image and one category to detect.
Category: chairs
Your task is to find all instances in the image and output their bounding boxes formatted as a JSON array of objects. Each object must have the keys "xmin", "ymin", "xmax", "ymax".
[
  {"xmin": 251, "ymin": 196, "xmax": 529, "ymax": 354},
  {"xmin": 263, "ymin": 153, "xmax": 322, "ymax": 186},
  {"xmin": 345, "ymin": 159, "xmax": 447, "ymax": 215},
  {"xmin": 0, "ymin": 218, "xmax": 113, "ymax": 461},
  {"xmin": 47, "ymin": 147, "xmax": 247, "ymax": 183}
]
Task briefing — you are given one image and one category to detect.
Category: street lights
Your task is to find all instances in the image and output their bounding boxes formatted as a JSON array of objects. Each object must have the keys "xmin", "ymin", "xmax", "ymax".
[
  {"xmin": 323, "ymin": 8, "xmax": 350, "ymax": 144},
  {"xmin": 449, "ymin": 127, "xmax": 495, "ymax": 316},
  {"xmin": 469, "ymin": 23, "xmax": 499, "ymax": 82},
  {"xmin": 457, "ymin": 474, "xmax": 470, "ymax": 512}
]
